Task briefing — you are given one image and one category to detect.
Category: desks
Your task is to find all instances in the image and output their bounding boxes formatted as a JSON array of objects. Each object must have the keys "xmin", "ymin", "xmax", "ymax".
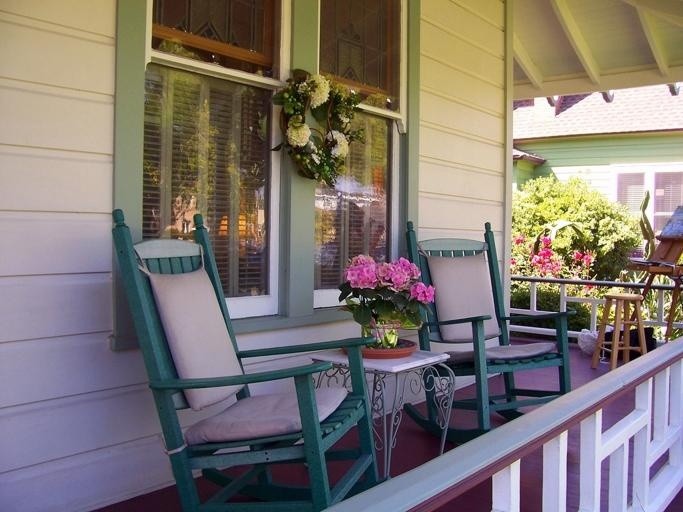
[{"xmin": 308, "ymin": 347, "xmax": 455, "ymax": 481}]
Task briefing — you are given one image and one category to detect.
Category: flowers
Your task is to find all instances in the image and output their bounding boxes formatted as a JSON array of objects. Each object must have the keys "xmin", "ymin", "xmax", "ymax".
[
  {"xmin": 267, "ymin": 68, "xmax": 368, "ymax": 189},
  {"xmin": 338, "ymin": 255, "xmax": 435, "ymax": 344}
]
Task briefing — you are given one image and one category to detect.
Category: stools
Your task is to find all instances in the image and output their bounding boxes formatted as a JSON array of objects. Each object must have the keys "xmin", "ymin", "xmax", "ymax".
[{"xmin": 590, "ymin": 291, "xmax": 648, "ymax": 371}]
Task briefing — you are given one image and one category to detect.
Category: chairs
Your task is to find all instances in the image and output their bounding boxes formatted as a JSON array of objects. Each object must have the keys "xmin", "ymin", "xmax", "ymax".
[
  {"xmin": 403, "ymin": 221, "xmax": 576, "ymax": 443},
  {"xmin": 110, "ymin": 208, "xmax": 381, "ymax": 512}
]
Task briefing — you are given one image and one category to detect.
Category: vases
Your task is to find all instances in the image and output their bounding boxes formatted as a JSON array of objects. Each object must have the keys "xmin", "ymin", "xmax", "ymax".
[{"xmin": 361, "ymin": 319, "xmax": 402, "ymax": 349}]
